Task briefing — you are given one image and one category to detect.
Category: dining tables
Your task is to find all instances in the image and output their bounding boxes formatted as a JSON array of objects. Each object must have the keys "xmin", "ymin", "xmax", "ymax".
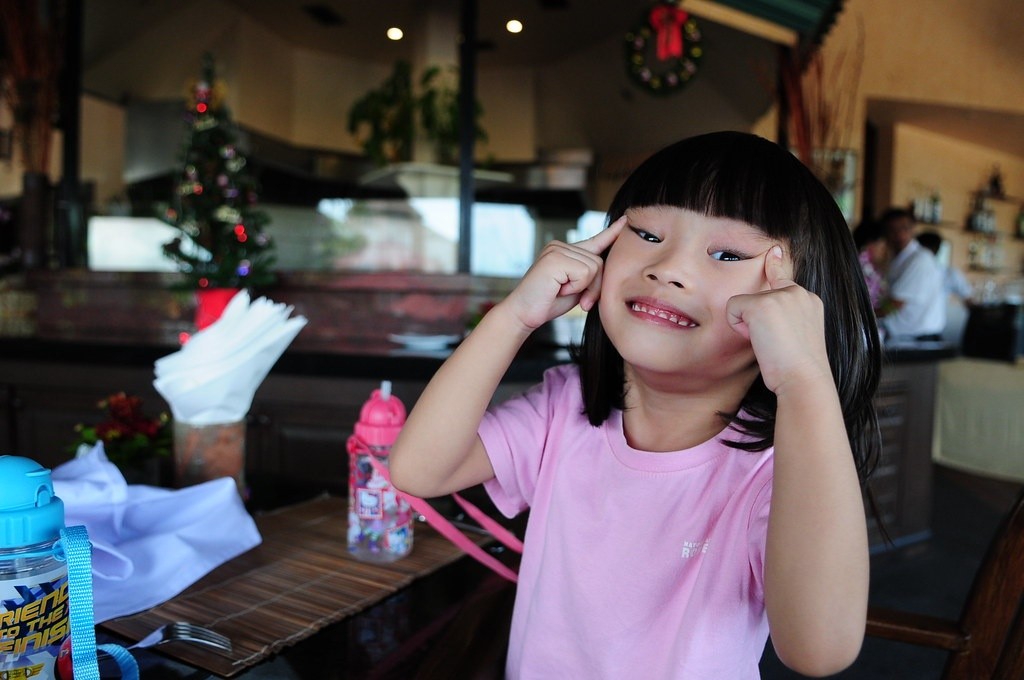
[
  {"xmin": 79, "ymin": 491, "xmax": 531, "ymax": 678},
  {"xmin": 1, "ymin": 323, "xmax": 578, "ymax": 517}
]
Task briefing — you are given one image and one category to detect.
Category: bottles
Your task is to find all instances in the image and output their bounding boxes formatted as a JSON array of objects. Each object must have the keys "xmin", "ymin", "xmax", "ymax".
[
  {"xmin": 0, "ymin": 455, "xmax": 74, "ymax": 680},
  {"xmin": 348, "ymin": 390, "xmax": 416, "ymax": 563}
]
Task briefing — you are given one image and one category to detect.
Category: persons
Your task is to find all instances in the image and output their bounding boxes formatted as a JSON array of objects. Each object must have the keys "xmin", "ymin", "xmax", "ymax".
[
  {"xmin": 385, "ymin": 128, "xmax": 881, "ymax": 680},
  {"xmin": 855, "ymin": 206, "xmax": 973, "ymax": 351}
]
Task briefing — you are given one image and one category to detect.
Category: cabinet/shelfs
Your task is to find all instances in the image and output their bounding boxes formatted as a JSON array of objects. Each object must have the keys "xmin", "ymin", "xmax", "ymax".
[{"xmin": 911, "ymin": 189, "xmax": 1024, "ymax": 272}]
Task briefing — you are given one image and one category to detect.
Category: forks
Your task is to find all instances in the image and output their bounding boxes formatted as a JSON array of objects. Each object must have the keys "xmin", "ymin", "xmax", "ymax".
[{"xmin": 99, "ymin": 623, "xmax": 232, "ymax": 654}]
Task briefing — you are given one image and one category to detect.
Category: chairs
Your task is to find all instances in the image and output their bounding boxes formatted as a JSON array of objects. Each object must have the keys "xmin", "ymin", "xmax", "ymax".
[{"xmin": 864, "ymin": 493, "xmax": 1021, "ymax": 679}]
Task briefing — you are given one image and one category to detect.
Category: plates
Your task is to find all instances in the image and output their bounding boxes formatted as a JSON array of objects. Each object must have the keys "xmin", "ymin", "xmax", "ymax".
[{"xmin": 388, "ymin": 331, "xmax": 459, "ymax": 351}]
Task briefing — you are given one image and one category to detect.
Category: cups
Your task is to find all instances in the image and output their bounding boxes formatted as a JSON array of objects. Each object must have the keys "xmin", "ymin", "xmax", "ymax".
[{"xmin": 174, "ymin": 417, "xmax": 249, "ymax": 502}]
